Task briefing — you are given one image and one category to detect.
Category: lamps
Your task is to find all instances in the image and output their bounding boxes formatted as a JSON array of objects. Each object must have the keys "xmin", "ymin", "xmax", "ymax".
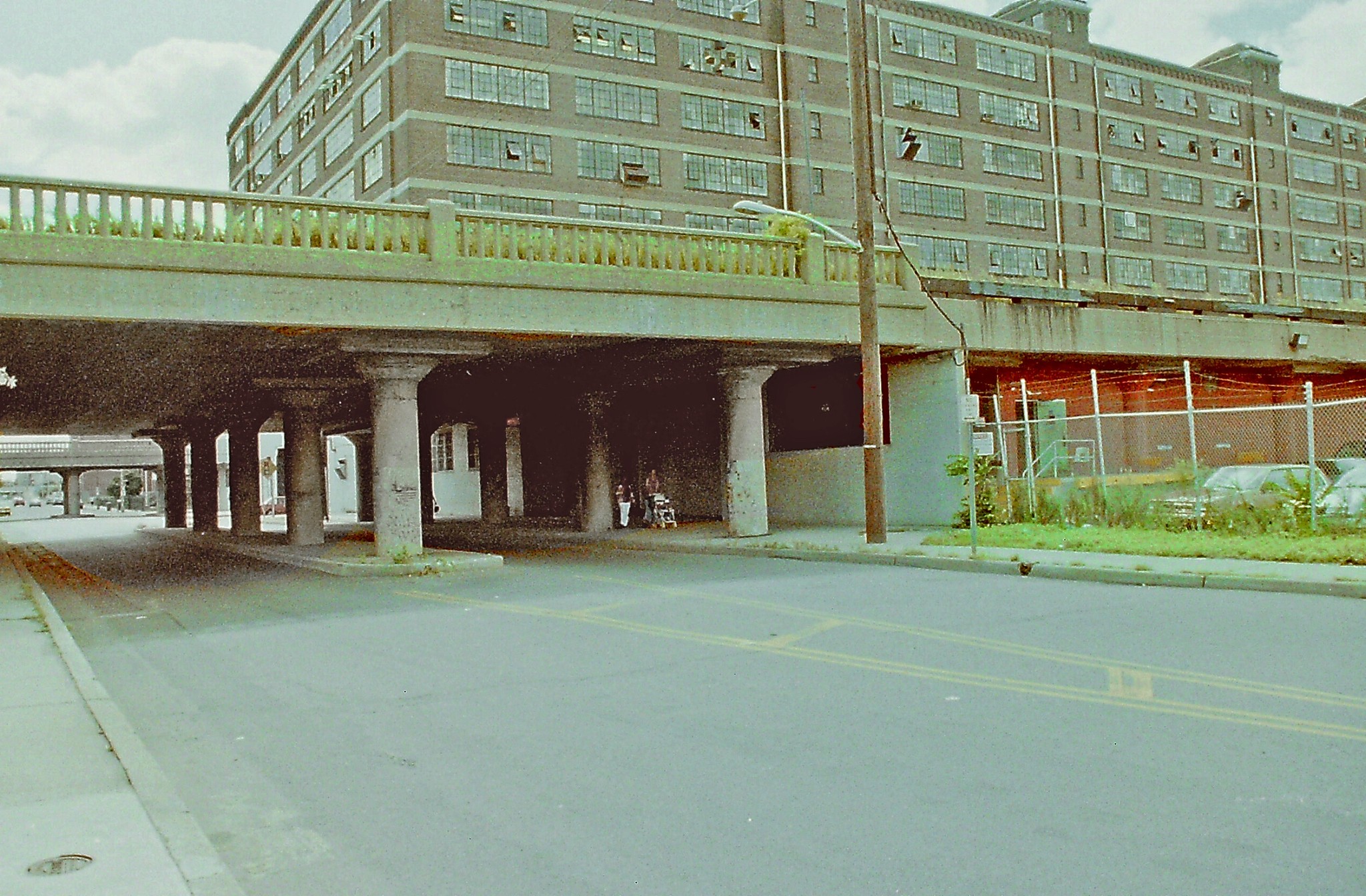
[{"xmin": 1289, "ymin": 333, "xmax": 1310, "ymax": 351}]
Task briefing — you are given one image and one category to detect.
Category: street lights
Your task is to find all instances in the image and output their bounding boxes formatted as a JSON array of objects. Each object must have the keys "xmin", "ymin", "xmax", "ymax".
[{"xmin": 734, "ymin": 200, "xmax": 889, "ymax": 547}]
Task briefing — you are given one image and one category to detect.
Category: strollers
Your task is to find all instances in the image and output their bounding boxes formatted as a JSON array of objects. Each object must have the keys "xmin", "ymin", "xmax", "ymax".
[{"xmin": 649, "ymin": 492, "xmax": 678, "ymax": 530}]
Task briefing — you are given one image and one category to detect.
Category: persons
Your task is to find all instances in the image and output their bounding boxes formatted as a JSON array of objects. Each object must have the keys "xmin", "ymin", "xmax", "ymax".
[{"xmin": 613, "ymin": 468, "xmax": 671, "ymax": 529}]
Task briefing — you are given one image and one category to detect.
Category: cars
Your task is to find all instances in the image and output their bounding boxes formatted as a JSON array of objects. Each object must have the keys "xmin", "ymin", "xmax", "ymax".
[
  {"xmin": 13, "ymin": 495, "xmax": 25, "ymax": 506},
  {"xmin": 30, "ymin": 497, "xmax": 42, "ymax": 507},
  {"xmin": 45, "ymin": 490, "xmax": 64, "ymax": 505},
  {"xmin": 79, "ymin": 498, "xmax": 84, "ymax": 509},
  {"xmin": 0, "ymin": 505, "xmax": 11, "ymax": 516}
]
